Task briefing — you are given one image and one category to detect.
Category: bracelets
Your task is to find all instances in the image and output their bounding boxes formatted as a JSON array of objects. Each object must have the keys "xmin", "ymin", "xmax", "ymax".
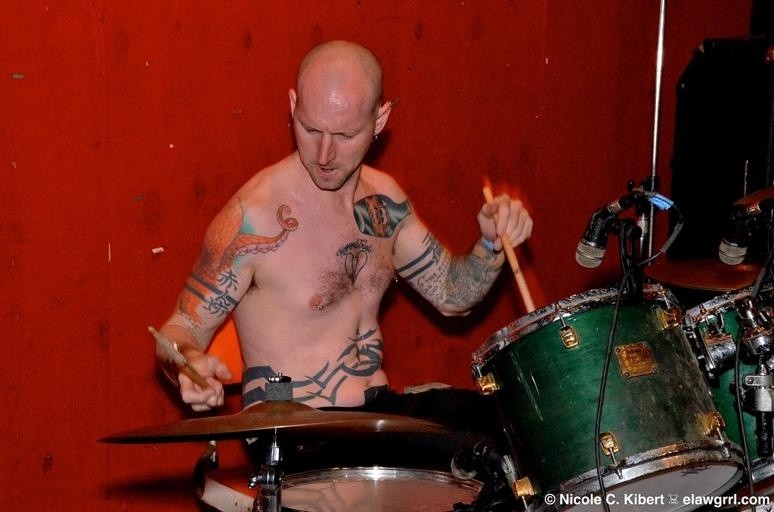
[{"xmin": 479, "ymin": 235, "xmax": 506, "ymax": 251}]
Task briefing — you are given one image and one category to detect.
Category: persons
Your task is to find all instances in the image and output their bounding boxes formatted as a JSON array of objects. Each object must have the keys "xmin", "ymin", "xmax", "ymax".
[
  {"xmin": 655, "ymin": 0, "xmax": 773, "ymax": 311},
  {"xmin": 152, "ymin": 36, "xmax": 536, "ymax": 486}
]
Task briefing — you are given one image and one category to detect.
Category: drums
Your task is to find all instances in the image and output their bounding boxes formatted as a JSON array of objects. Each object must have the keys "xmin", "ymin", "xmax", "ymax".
[
  {"xmin": 257, "ymin": 383, "xmax": 498, "ymax": 508},
  {"xmin": 469, "ymin": 283, "xmax": 745, "ymax": 510},
  {"xmin": 684, "ymin": 287, "xmax": 773, "ymax": 485}
]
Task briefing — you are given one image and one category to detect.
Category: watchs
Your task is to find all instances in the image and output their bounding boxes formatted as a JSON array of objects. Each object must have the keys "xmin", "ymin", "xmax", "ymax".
[{"xmin": 162, "ymin": 340, "xmax": 202, "ymax": 389}]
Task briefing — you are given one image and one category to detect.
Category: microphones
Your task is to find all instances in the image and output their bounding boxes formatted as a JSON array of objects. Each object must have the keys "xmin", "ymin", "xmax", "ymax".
[
  {"xmin": 718, "ymin": 198, "xmax": 767, "ymax": 265},
  {"xmin": 576, "ymin": 188, "xmax": 643, "ymax": 267}
]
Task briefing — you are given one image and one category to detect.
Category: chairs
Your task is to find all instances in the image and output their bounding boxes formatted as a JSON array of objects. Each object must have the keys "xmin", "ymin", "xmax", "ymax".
[{"xmin": 194, "ymin": 320, "xmax": 260, "ymax": 512}]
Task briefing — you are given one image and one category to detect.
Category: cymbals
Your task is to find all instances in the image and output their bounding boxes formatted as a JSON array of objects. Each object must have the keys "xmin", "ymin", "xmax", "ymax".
[{"xmin": 97, "ymin": 401, "xmax": 448, "ymax": 443}]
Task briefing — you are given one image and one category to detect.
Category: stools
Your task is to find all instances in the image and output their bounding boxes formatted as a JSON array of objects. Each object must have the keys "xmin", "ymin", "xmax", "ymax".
[{"xmin": 641, "ymin": 226, "xmax": 767, "ymax": 294}]
